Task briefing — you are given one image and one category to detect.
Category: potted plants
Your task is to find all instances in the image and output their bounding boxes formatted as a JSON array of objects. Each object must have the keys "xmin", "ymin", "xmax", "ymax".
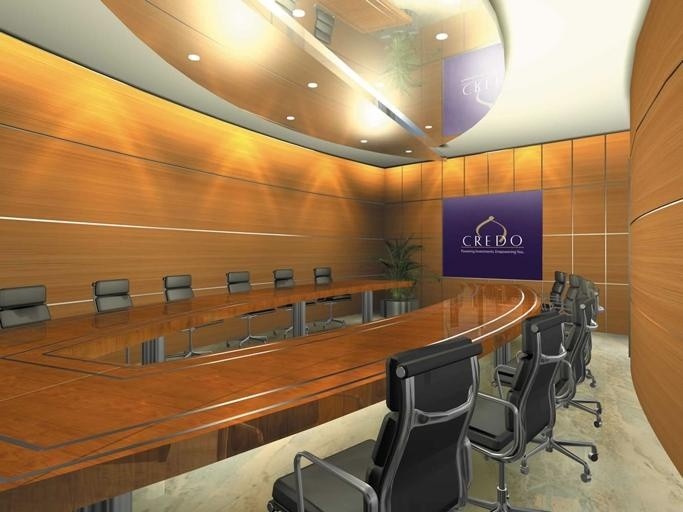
[
  {"xmin": 371, "ymin": 10, "xmax": 442, "ymax": 98},
  {"xmin": 376, "ymin": 233, "xmax": 442, "ymax": 317}
]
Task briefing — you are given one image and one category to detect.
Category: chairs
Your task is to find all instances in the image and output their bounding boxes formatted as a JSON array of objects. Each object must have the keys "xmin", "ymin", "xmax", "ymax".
[{"xmin": 270, "ymin": 0, "xmax": 335, "ymax": 45}]
